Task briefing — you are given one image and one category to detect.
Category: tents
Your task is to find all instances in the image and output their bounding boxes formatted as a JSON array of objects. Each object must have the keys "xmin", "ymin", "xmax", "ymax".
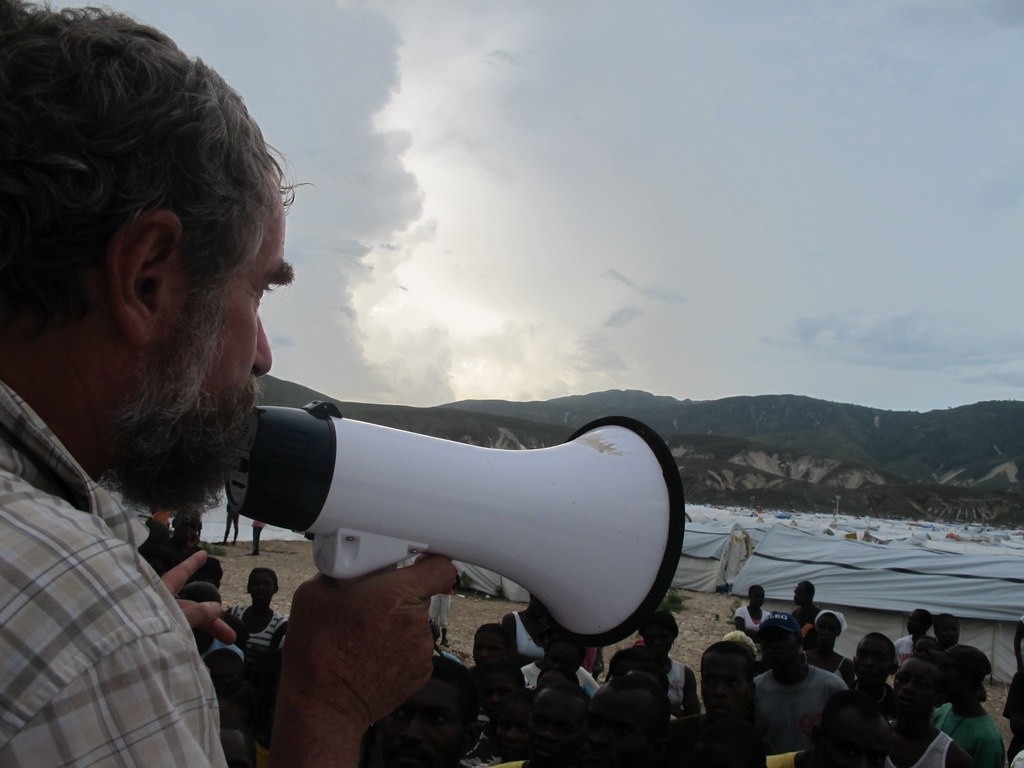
[{"xmin": 451, "ymin": 504, "xmax": 1024, "ymax": 686}]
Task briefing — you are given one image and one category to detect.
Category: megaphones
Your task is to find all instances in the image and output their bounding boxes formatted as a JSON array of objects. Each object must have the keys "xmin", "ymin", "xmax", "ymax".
[{"xmin": 225, "ymin": 400, "xmax": 686, "ymax": 647}]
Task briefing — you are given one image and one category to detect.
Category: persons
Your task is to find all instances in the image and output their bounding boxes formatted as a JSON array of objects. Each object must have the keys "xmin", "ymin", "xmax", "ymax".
[
  {"xmin": 137, "ymin": 503, "xmax": 1024, "ymax": 768},
  {"xmin": 0, "ymin": 0, "xmax": 460, "ymax": 768}
]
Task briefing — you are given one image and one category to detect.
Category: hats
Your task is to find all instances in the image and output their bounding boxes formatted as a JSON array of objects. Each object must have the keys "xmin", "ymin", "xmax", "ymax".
[
  {"xmin": 758, "ymin": 609, "xmax": 803, "ymax": 639},
  {"xmin": 814, "ymin": 606, "xmax": 848, "ymax": 636},
  {"xmin": 146, "ymin": 512, "xmax": 170, "ymax": 530}
]
